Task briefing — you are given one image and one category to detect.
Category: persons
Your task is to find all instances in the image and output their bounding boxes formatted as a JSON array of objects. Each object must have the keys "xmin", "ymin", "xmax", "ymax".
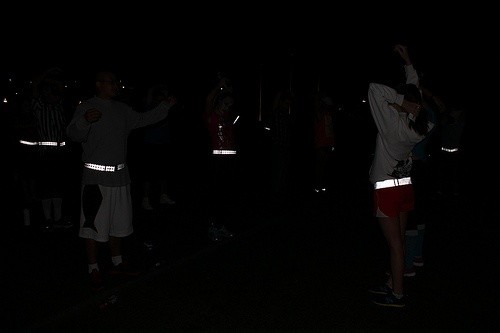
[
  {"xmin": 271, "ymin": 90, "xmax": 296, "ymax": 191},
  {"xmin": 28, "ymin": 62, "xmax": 76, "ymax": 232},
  {"xmin": 66, "ymin": 70, "xmax": 176, "ymax": 292},
  {"xmin": 368, "ymin": 42, "xmax": 435, "ymax": 307},
  {"xmin": 204, "ymin": 73, "xmax": 244, "ymax": 242}
]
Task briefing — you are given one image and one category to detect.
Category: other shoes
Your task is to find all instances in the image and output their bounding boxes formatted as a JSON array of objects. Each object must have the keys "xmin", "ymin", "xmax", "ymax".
[
  {"xmin": 86, "ymin": 270, "xmax": 105, "ymax": 295},
  {"xmin": 112, "ymin": 268, "xmax": 131, "ymax": 284},
  {"xmin": 367, "ymin": 284, "xmax": 406, "ymax": 308}
]
